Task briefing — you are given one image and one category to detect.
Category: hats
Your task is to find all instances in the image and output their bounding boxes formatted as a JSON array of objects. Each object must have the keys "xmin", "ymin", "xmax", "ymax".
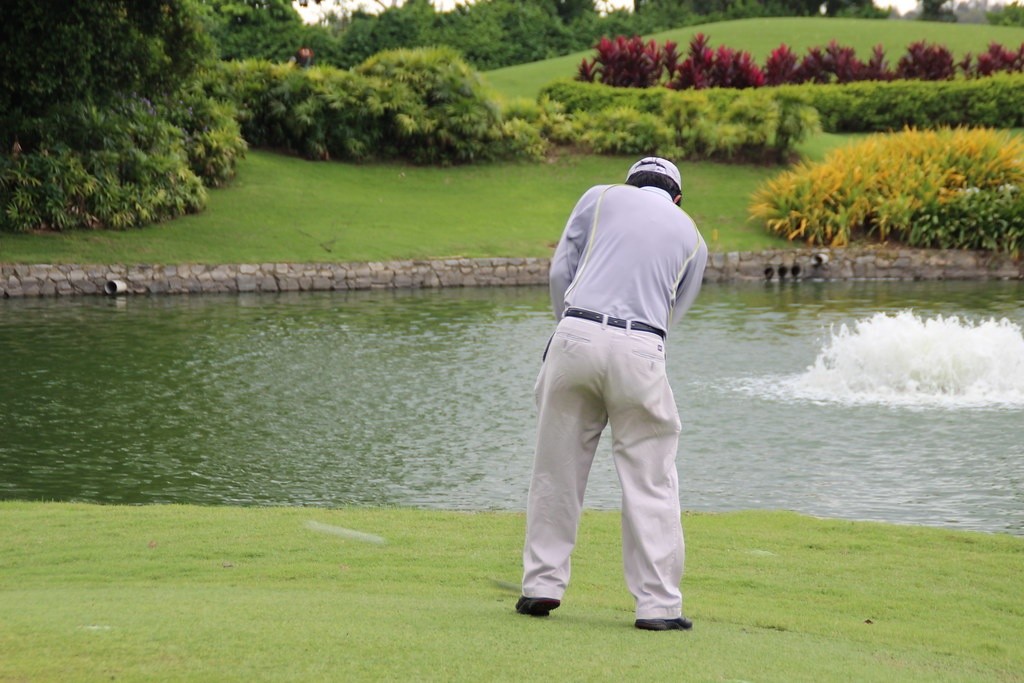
[{"xmin": 626, "ymin": 157, "xmax": 682, "ymax": 191}]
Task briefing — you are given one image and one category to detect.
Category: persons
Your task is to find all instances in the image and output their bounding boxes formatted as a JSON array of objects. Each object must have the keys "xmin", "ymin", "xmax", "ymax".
[{"xmin": 515, "ymin": 158, "xmax": 708, "ymax": 631}]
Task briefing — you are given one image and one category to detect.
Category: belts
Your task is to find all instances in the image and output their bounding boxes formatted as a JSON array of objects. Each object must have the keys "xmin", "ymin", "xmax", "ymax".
[{"xmin": 565, "ymin": 307, "xmax": 663, "ymax": 339}]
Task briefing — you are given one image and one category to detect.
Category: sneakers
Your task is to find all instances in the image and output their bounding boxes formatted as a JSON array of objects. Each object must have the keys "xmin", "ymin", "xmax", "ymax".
[
  {"xmin": 635, "ymin": 616, "xmax": 692, "ymax": 631},
  {"xmin": 515, "ymin": 595, "xmax": 560, "ymax": 616}
]
